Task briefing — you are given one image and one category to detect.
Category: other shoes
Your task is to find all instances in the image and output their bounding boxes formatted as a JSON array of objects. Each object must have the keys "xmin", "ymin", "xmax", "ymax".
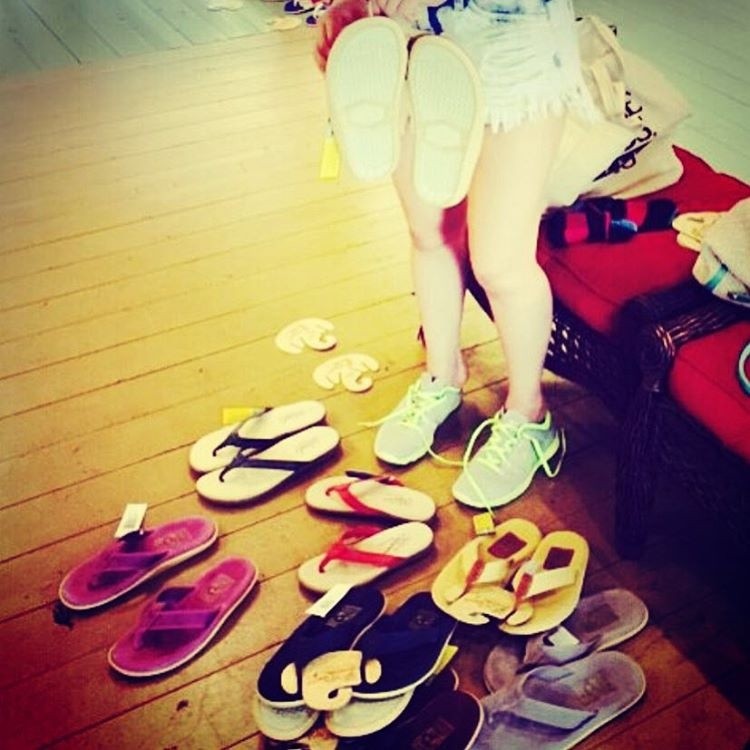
[
  {"xmin": 283, "ymin": 0, "xmax": 327, "ymax": 13},
  {"xmin": 304, "ymin": 5, "xmax": 327, "ymax": 27},
  {"xmin": 408, "ymin": 34, "xmax": 484, "ymax": 210},
  {"xmin": 252, "ymin": 583, "xmax": 485, "ymax": 750},
  {"xmin": 325, "ymin": 16, "xmax": 408, "ymax": 184}
]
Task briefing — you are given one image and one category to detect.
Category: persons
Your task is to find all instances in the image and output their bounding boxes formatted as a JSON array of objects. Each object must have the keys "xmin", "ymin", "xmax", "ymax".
[{"xmin": 315, "ymin": 0, "xmax": 584, "ymax": 510}]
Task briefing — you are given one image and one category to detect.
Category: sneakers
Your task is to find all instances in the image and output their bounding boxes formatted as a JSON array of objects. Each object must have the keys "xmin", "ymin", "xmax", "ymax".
[
  {"xmin": 373, "ymin": 371, "xmax": 463, "ymax": 466},
  {"xmin": 451, "ymin": 406, "xmax": 561, "ymax": 510}
]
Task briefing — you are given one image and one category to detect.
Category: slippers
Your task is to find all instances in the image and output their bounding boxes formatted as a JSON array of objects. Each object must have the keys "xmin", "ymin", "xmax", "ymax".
[
  {"xmin": 298, "ymin": 522, "xmax": 436, "ymax": 593},
  {"xmin": 431, "ymin": 517, "xmax": 542, "ymax": 624},
  {"xmin": 189, "ymin": 399, "xmax": 327, "ymax": 471},
  {"xmin": 58, "ymin": 516, "xmax": 219, "ymax": 611},
  {"xmin": 305, "ymin": 470, "xmax": 436, "ymax": 521},
  {"xmin": 194, "ymin": 426, "xmax": 342, "ymax": 505},
  {"xmin": 108, "ymin": 558, "xmax": 260, "ymax": 678},
  {"xmin": 466, "ymin": 651, "xmax": 646, "ymax": 750},
  {"xmin": 483, "ymin": 588, "xmax": 649, "ymax": 694},
  {"xmin": 495, "ymin": 532, "xmax": 589, "ymax": 634}
]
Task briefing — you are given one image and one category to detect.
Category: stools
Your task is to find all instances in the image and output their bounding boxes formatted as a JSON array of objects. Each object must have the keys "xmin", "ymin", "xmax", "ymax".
[{"xmin": 465, "ymin": 142, "xmax": 750, "ymax": 565}]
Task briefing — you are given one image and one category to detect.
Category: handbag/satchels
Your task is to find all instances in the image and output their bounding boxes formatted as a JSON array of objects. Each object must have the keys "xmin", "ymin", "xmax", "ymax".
[{"xmin": 542, "ymin": 13, "xmax": 684, "ymax": 210}]
